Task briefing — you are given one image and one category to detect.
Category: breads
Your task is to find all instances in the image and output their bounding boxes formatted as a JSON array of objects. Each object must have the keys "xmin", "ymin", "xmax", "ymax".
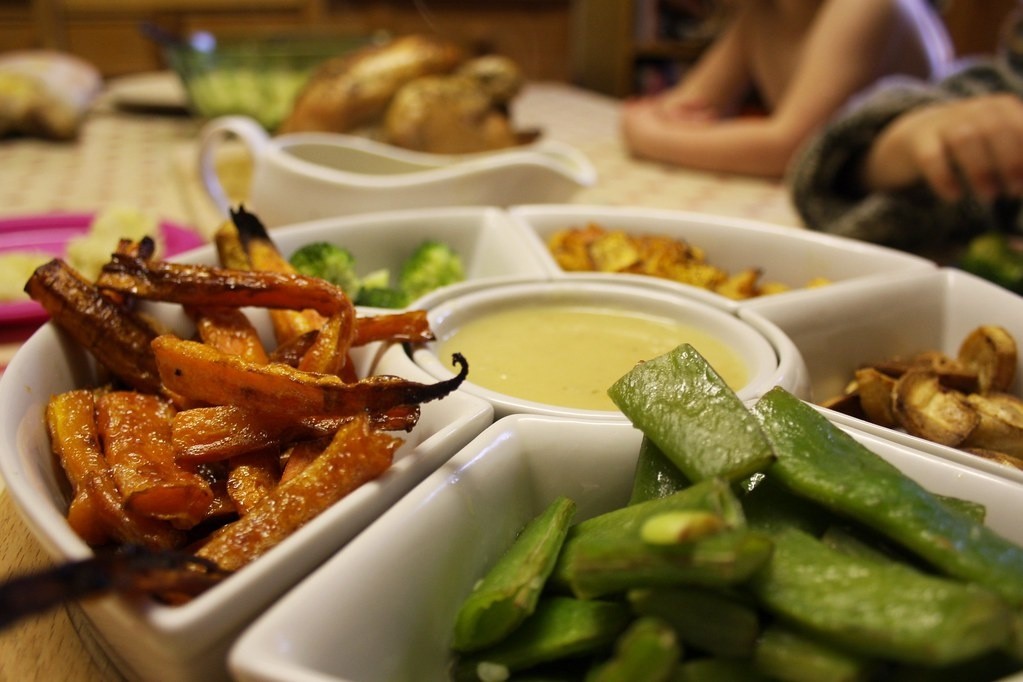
[{"xmin": 278, "ymin": 32, "xmax": 524, "ymax": 153}]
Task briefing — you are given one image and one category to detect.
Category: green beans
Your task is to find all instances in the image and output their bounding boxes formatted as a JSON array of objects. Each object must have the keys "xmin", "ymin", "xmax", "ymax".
[{"xmin": 428, "ymin": 346, "xmax": 1023, "ymax": 682}]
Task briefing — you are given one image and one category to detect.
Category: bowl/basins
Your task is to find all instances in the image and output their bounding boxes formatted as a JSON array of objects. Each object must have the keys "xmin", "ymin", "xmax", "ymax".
[
  {"xmin": 155, "ymin": 36, "xmax": 390, "ymax": 129},
  {"xmin": 407, "ymin": 280, "xmax": 776, "ymax": 423}
]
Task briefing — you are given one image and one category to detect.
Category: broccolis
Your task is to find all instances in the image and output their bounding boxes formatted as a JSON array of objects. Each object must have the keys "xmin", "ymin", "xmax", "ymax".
[{"xmin": 291, "ymin": 239, "xmax": 464, "ymax": 309}]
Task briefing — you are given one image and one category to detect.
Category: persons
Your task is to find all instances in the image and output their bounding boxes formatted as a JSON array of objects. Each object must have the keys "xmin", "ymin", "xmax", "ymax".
[
  {"xmin": 621, "ymin": 0, "xmax": 951, "ymax": 186},
  {"xmin": 789, "ymin": 12, "xmax": 1023, "ymax": 261}
]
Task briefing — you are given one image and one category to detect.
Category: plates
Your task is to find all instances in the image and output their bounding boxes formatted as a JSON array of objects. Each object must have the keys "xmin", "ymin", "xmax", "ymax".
[
  {"xmin": 0, "ymin": 204, "xmax": 1023, "ymax": 681},
  {"xmin": 0, "ymin": 211, "xmax": 207, "ymax": 330}
]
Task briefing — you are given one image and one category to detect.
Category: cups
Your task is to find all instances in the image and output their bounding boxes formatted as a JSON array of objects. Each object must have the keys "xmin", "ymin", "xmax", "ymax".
[{"xmin": 200, "ymin": 116, "xmax": 598, "ymax": 230}]
